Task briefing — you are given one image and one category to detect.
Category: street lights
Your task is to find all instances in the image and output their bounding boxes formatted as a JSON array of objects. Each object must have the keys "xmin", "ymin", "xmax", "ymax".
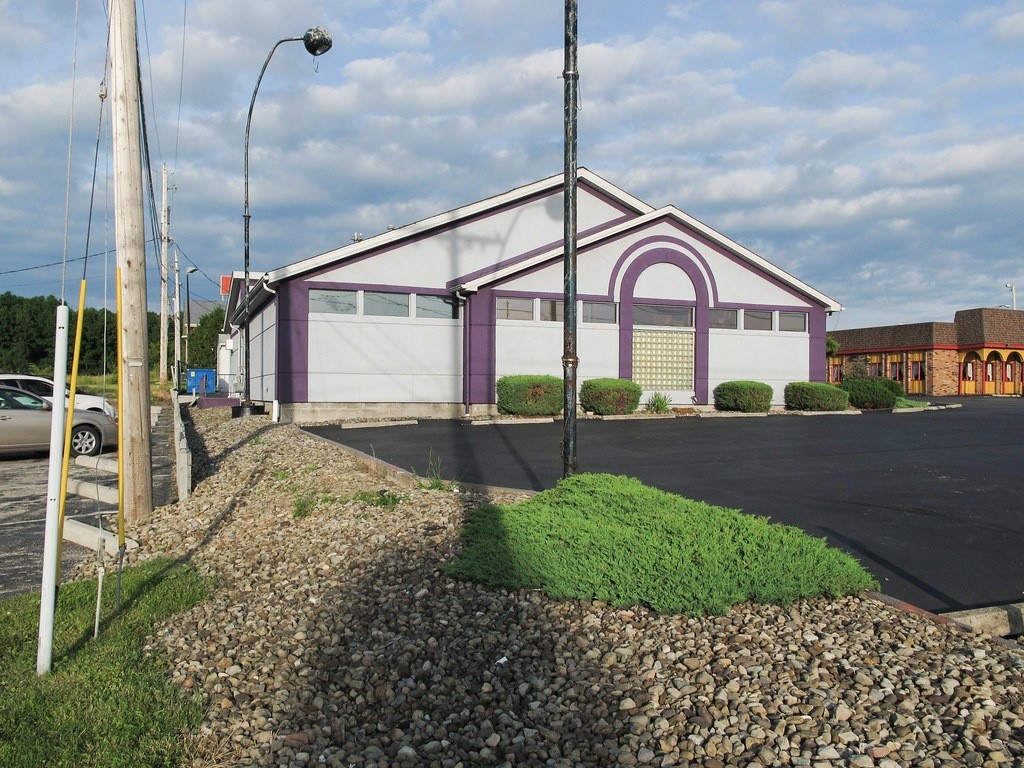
[
  {"xmin": 1005, "ymin": 283, "xmax": 1016, "ymax": 309},
  {"xmin": 233, "ymin": 25, "xmax": 333, "ymax": 428},
  {"xmin": 184, "ymin": 267, "xmax": 199, "ymax": 367}
]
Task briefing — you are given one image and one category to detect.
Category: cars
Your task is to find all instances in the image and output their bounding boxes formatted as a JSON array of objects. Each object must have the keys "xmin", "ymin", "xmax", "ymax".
[
  {"xmin": 0, "ymin": 373, "xmax": 116, "ymax": 418},
  {"xmin": 0, "ymin": 384, "xmax": 118, "ymax": 458}
]
